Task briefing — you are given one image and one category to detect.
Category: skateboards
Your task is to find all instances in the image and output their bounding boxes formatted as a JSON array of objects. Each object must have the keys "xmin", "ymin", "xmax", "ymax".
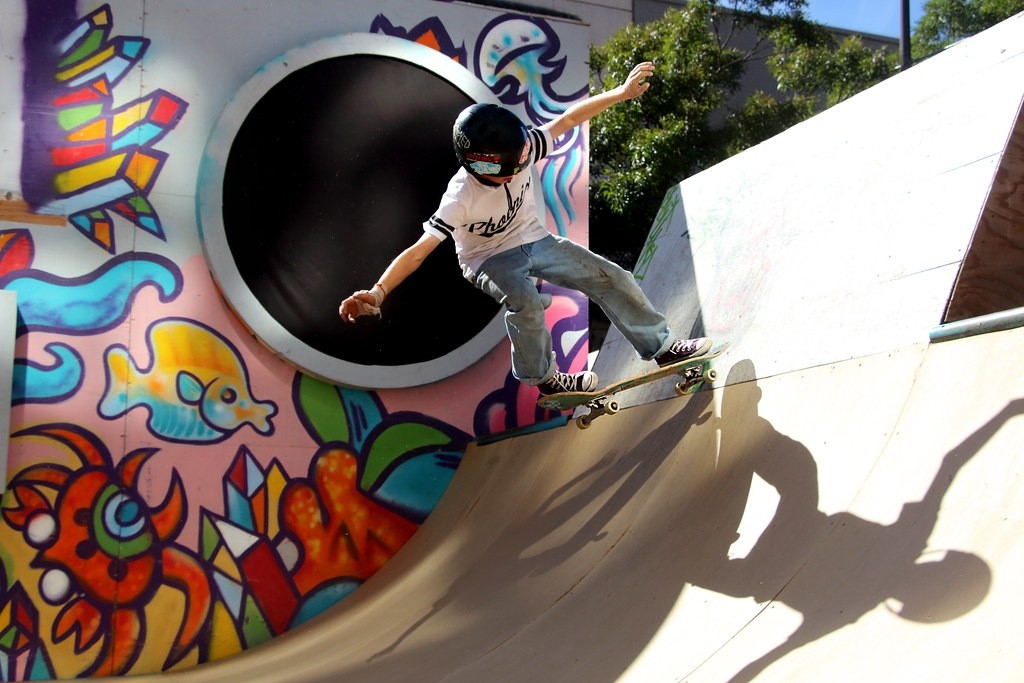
[{"xmin": 534, "ymin": 339, "xmax": 732, "ymax": 430}]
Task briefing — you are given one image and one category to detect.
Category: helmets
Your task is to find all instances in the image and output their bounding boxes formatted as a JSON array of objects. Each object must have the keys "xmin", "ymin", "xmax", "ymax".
[{"xmin": 452, "ymin": 103, "xmax": 533, "ymax": 188}]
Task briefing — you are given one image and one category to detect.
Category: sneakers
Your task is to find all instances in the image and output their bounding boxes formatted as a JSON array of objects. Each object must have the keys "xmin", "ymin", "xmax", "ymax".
[
  {"xmin": 654, "ymin": 337, "xmax": 713, "ymax": 369},
  {"xmin": 537, "ymin": 370, "xmax": 598, "ymax": 395}
]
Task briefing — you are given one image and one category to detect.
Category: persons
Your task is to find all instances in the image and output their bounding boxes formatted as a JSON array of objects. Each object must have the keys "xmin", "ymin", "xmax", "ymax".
[{"xmin": 339, "ymin": 62, "xmax": 712, "ymax": 395}]
